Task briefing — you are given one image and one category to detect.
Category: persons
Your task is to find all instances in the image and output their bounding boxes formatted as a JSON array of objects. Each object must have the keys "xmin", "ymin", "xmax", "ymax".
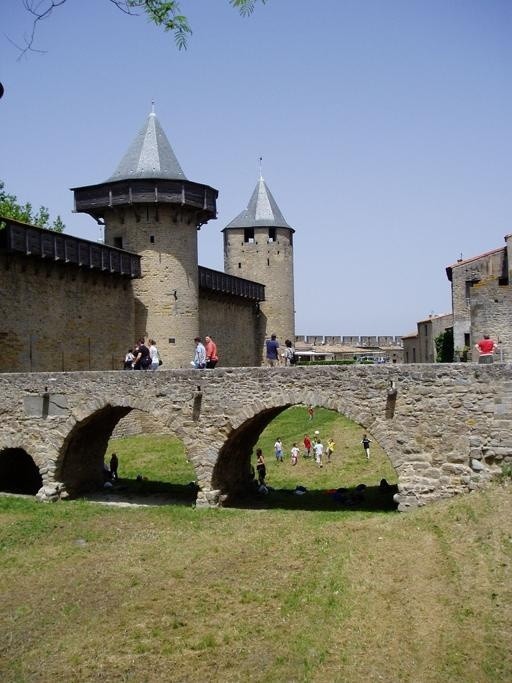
[
  {"xmin": 102, "ymin": 447, "xmax": 126, "ymax": 483},
  {"xmin": 474, "ymin": 332, "xmax": 499, "ymax": 364},
  {"xmin": 243, "ymin": 400, "xmax": 396, "ymax": 516},
  {"xmin": 111, "ymin": 331, "xmax": 308, "ymax": 369}
]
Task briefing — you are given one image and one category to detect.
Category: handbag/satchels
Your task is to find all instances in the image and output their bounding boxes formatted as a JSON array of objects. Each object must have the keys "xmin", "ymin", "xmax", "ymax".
[
  {"xmin": 159, "ymin": 359, "xmax": 162, "ymax": 365},
  {"xmin": 141, "ymin": 356, "xmax": 152, "ymax": 369}
]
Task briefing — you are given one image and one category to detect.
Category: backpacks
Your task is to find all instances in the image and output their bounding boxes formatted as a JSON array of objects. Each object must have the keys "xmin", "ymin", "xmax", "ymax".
[{"xmin": 287, "ymin": 348, "xmax": 300, "ymax": 364}]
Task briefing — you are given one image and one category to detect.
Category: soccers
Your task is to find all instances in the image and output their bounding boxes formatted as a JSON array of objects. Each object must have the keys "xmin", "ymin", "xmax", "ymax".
[{"xmin": 314, "ymin": 431, "xmax": 319, "ymax": 434}]
